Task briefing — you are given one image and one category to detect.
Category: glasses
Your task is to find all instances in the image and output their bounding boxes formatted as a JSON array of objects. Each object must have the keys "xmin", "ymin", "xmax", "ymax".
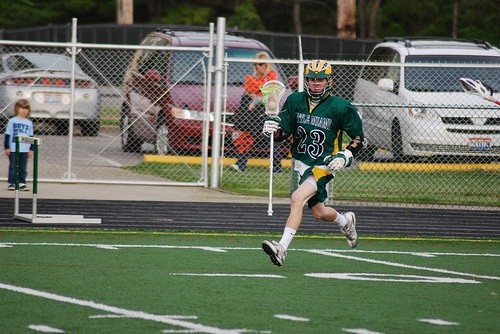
[{"xmin": 254, "ymin": 63, "xmax": 265, "ymax": 66}]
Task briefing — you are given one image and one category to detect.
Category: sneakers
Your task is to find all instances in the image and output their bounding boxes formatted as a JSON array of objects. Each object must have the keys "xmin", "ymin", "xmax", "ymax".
[
  {"xmin": 338, "ymin": 211, "xmax": 358, "ymax": 248},
  {"xmin": 262, "ymin": 239, "xmax": 288, "ymax": 266}
]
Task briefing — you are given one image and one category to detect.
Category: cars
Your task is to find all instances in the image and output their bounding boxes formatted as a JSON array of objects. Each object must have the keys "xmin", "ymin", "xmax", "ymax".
[
  {"xmin": 118, "ymin": 27, "xmax": 295, "ymax": 161},
  {"xmin": 0, "ymin": 52, "xmax": 101, "ymax": 138},
  {"xmin": 352, "ymin": 36, "xmax": 500, "ymax": 163}
]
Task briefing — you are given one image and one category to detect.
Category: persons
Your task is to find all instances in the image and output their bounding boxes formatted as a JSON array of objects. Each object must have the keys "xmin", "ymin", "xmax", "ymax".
[
  {"xmin": 4, "ymin": 99, "xmax": 33, "ymax": 191},
  {"xmin": 262, "ymin": 59, "xmax": 365, "ymax": 267},
  {"xmin": 230, "ymin": 53, "xmax": 283, "ymax": 175}
]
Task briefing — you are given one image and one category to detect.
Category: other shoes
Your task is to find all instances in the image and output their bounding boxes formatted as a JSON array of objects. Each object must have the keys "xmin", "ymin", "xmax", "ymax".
[
  {"xmin": 19, "ymin": 182, "xmax": 30, "ymax": 191},
  {"xmin": 230, "ymin": 164, "xmax": 242, "ymax": 172},
  {"xmin": 8, "ymin": 182, "xmax": 16, "ymax": 190}
]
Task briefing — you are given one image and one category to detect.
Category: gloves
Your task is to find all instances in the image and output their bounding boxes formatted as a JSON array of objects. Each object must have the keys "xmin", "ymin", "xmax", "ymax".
[
  {"xmin": 261, "ymin": 115, "xmax": 282, "ymax": 142},
  {"xmin": 323, "ymin": 149, "xmax": 353, "ymax": 171}
]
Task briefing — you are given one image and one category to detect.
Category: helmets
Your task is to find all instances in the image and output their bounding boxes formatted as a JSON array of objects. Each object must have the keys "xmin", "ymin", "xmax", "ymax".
[{"xmin": 305, "ymin": 59, "xmax": 333, "ymax": 103}]
